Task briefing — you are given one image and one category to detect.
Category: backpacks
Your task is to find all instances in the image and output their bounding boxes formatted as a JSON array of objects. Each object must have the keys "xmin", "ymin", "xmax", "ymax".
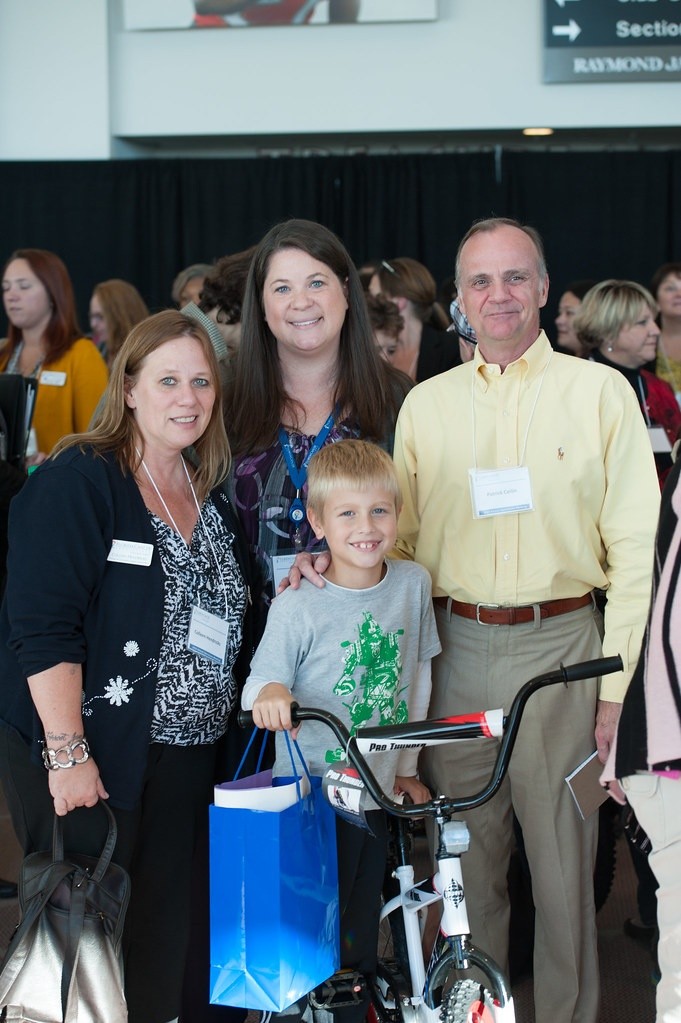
[{"xmin": 0, "ymin": 798, "xmax": 131, "ymax": 1023}]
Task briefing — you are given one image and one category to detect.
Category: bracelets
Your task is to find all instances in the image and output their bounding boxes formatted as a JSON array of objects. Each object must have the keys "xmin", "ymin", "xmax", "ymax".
[{"xmin": 41, "ymin": 737, "xmax": 91, "ymax": 769}]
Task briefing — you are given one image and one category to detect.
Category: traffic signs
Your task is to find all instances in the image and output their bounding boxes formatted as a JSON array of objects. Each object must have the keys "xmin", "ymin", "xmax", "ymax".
[{"xmin": 537, "ymin": 0, "xmax": 681, "ymax": 84}]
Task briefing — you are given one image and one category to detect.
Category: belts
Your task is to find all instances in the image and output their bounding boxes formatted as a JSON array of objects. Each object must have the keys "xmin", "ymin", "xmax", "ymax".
[{"xmin": 432, "ymin": 590, "xmax": 602, "ymax": 626}]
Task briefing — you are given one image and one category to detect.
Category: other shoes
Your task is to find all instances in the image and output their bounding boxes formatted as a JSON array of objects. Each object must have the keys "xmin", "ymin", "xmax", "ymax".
[
  {"xmin": 651, "ymin": 969, "xmax": 661, "ymax": 986},
  {"xmin": 0, "ymin": 879, "xmax": 18, "ymax": 899},
  {"xmin": 623, "ymin": 917, "xmax": 656, "ymax": 938}
]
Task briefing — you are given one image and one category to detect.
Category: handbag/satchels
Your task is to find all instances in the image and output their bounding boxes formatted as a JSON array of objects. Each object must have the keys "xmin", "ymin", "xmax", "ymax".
[{"xmin": 204, "ymin": 725, "xmax": 340, "ymax": 1014}]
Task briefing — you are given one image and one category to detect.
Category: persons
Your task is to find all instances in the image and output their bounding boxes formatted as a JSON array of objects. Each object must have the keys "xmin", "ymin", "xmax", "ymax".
[
  {"xmin": 239, "ymin": 439, "xmax": 445, "ymax": 1023},
  {"xmin": 273, "ymin": 219, "xmax": 664, "ymax": 1023},
  {"xmin": 0, "ymin": 247, "xmax": 681, "ymax": 1023},
  {"xmin": 0, "ymin": 308, "xmax": 268, "ymax": 1023},
  {"xmin": 225, "ymin": 217, "xmax": 417, "ymax": 592}
]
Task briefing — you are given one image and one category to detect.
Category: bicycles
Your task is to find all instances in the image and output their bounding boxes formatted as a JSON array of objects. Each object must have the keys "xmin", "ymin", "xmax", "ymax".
[{"xmin": 231, "ymin": 653, "xmax": 628, "ymax": 1023}]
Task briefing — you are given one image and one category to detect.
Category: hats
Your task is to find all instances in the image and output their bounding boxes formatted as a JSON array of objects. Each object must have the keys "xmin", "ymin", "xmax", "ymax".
[{"xmin": 446, "ymin": 298, "xmax": 477, "ymax": 344}]
